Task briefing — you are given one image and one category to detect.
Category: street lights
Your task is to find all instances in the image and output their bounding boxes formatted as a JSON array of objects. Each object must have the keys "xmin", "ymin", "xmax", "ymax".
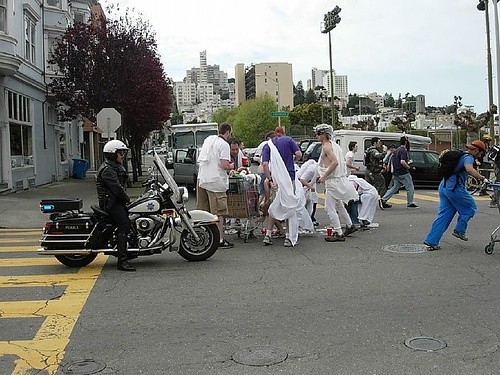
[
  {"xmin": 477, "ymin": 0, "xmax": 494, "ymax": 142},
  {"xmin": 320, "ymin": 4, "xmax": 343, "ymax": 131}
]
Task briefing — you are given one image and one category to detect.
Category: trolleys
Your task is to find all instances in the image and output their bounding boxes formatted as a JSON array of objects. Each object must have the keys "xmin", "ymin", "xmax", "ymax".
[
  {"xmin": 482, "ymin": 179, "xmax": 500, "ymax": 256},
  {"xmin": 222, "ymin": 170, "xmax": 264, "ymax": 242}
]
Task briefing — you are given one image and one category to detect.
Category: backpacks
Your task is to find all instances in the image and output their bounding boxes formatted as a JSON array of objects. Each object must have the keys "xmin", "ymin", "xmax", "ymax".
[
  {"xmin": 436, "ymin": 148, "xmax": 474, "ymax": 192},
  {"xmin": 362, "ymin": 148, "xmax": 376, "ymax": 166}
]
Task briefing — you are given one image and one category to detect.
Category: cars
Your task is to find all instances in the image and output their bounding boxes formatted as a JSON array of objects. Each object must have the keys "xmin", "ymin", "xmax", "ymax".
[
  {"xmin": 382, "ymin": 148, "xmax": 446, "ymax": 189},
  {"xmin": 335, "ymin": 129, "xmax": 432, "ymax": 180},
  {"xmin": 241, "ymin": 148, "xmax": 261, "ymax": 168},
  {"xmin": 299, "ymin": 138, "xmax": 322, "ymax": 165}
]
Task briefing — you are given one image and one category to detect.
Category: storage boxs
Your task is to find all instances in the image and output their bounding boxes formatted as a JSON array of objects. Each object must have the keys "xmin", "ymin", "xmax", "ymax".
[
  {"xmin": 50, "ymin": 217, "xmax": 94, "ymax": 234},
  {"xmin": 40, "ymin": 197, "xmax": 83, "ymax": 212}
]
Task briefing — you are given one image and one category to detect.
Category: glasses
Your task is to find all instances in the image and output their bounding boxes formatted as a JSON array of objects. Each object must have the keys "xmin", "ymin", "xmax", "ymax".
[{"xmin": 117, "ymin": 150, "xmax": 127, "ymax": 156}]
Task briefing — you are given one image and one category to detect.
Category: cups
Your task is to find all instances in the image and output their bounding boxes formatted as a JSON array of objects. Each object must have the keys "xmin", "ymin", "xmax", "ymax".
[
  {"xmin": 327, "ymin": 228, "xmax": 332, "ymax": 236},
  {"xmin": 242, "ymin": 157, "xmax": 248, "ymax": 165},
  {"xmin": 229, "ymin": 161, "xmax": 233, "ymax": 170}
]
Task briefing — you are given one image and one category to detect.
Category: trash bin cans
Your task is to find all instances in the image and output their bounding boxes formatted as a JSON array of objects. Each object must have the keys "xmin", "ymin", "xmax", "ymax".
[{"xmin": 70, "ymin": 158, "xmax": 89, "ymax": 180}]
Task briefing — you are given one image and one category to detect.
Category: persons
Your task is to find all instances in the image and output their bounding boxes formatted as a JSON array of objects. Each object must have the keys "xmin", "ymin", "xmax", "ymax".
[
  {"xmin": 477, "ymin": 134, "xmax": 493, "ymax": 196},
  {"xmin": 97, "ymin": 140, "xmax": 136, "ymax": 271},
  {"xmin": 197, "ymin": 123, "xmax": 420, "ymax": 249},
  {"xmin": 423, "ymin": 140, "xmax": 491, "ymax": 250}
]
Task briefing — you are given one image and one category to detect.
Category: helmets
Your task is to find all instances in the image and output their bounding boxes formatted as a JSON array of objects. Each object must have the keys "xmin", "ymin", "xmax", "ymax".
[{"xmin": 103, "ymin": 140, "xmax": 129, "ymax": 153}]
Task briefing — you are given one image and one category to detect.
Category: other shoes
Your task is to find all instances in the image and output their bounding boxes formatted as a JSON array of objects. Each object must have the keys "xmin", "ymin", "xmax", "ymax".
[
  {"xmin": 271, "ymin": 230, "xmax": 286, "ymax": 239},
  {"xmin": 423, "ymin": 240, "xmax": 441, "ymax": 250},
  {"xmin": 224, "ymin": 227, "xmax": 238, "ymax": 235},
  {"xmin": 262, "ymin": 235, "xmax": 273, "ymax": 244},
  {"xmin": 283, "ymin": 238, "xmax": 299, "ymax": 247},
  {"xmin": 406, "ymin": 204, "xmax": 419, "ymax": 209},
  {"xmin": 378, "ymin": 198, "xmax": 392, "ymax": 210},
  {"xmin": 298, "ymin": 228, "xmax": 315, "ymax": 237},
  {"xmin": 451, "ymin": 230, "xmax": 469, "ymax": 241}
]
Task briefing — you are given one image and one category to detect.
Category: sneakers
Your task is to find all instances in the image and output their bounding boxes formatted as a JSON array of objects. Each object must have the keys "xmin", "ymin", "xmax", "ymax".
[
  {"xmin": 325, "ymin": 231, "xmax": 346, "ymax": 242},
  {"xmin": 217, "ymin": 238, "xmax": 235, "ymax": 249},
  {"xmin": 343, "ymin": 224, "xmax": 358, "ymax": 236}
]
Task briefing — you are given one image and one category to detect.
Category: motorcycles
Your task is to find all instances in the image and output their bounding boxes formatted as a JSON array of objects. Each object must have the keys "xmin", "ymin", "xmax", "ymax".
[{"xmin": 39, "ymin": 152, "xmax": 221, "ymax": 268}]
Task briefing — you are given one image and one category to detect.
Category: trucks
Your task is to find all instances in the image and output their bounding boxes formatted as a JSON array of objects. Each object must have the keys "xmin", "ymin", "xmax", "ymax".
[{"xmin": 167, "ymin": 122, "xmax": 220, "ymax": 165}]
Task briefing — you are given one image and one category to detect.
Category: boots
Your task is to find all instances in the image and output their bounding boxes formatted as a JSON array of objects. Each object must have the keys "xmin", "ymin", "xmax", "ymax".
[{"xmin": 116, "ymin": 233, "xmax": 136, "ymax": 272}]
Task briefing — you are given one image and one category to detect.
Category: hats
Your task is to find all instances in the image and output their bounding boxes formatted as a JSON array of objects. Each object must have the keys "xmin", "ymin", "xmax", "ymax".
[{"xmin": 466, "ymin": 140, "xmax": 485, "ymax": 151}]
